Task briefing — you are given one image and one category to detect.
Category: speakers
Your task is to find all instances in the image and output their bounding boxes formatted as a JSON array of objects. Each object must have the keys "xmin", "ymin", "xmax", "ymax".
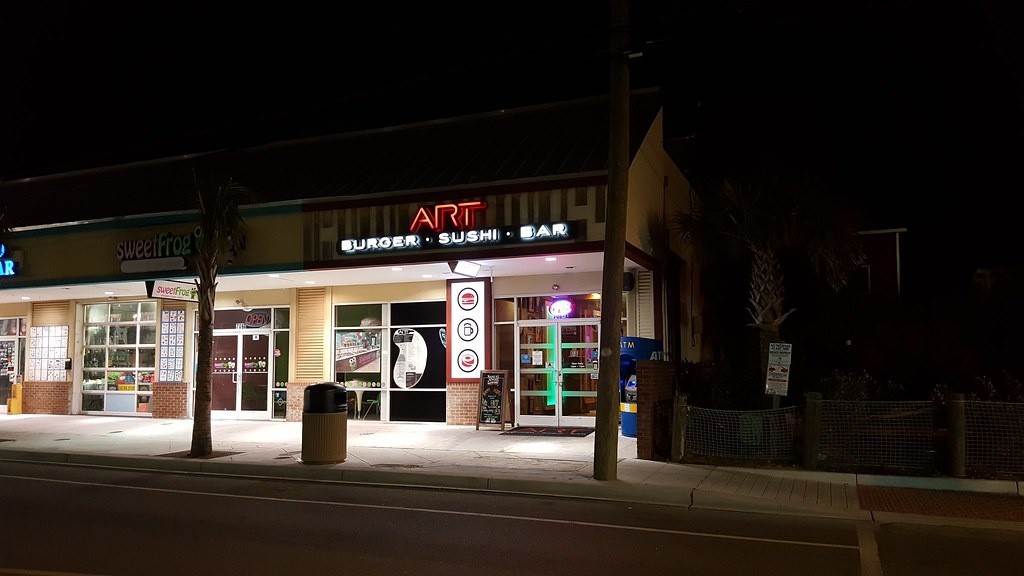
[{"xmin": 623, "ymin": 271, "xmax": 635, "ymax": 291}]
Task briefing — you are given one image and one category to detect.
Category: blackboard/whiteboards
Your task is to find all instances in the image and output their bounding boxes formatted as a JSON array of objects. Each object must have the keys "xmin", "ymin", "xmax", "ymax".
[{"xmin": 477, "ymin": 369, "xmax": 508, "ymax": 427}]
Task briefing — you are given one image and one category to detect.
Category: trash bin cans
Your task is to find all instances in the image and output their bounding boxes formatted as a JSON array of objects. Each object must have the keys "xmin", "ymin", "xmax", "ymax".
[{"xmin": 301, "ymin": 382, "xmax": 347, "ymax": 465}]
